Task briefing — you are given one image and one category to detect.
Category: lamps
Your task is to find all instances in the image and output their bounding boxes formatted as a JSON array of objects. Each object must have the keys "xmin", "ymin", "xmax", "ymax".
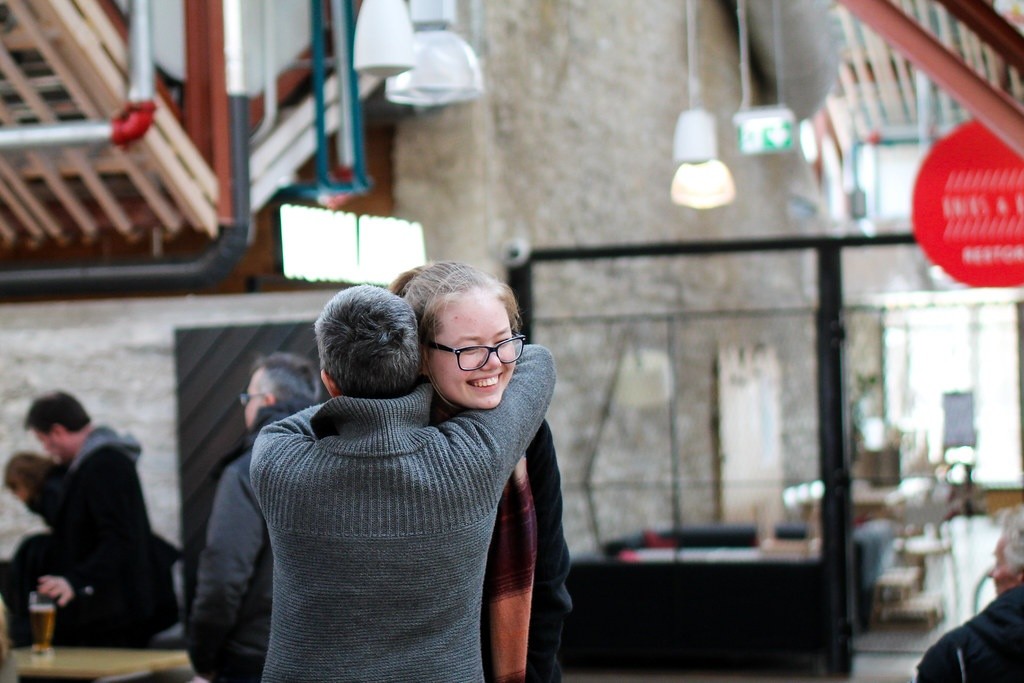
[
  {"xmin": 384, "ymin": 0, "xmax": 483, "ymax": 107},
  {"xmin": 353, "ymin": 0, "xmax": 417, "ymax": 77},
  {"xmin": 672, "ymin": 161, "xmax": 736, "ymax": 211},
  {"xmin": 672, "ymin": 0, "xmax": 718, "ymax": 163}
]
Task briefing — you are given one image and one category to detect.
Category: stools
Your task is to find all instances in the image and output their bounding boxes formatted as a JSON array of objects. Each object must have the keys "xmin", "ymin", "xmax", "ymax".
[{"xmin": 898, "ymin": 535, "xmax": 962, "ymax": 618}]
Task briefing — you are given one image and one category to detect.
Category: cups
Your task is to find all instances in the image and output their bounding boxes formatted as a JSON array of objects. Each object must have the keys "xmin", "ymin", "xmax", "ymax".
[{"xmin": 28, "ymin": 591, "xmax": 55, "ymax": 656}]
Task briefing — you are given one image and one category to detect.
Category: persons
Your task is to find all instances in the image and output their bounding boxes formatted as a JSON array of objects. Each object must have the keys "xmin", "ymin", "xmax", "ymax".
[
  {"xmin": 3, "ymin": 450, "xmax": 178, "ymax": 655},
  {"xmin": 245, "ymin": 283, "xmax": 555, "ymax": 683},
  {"xmin": 916, "ymin": 507, "xmax": 1024, "ymax": 683},
  {"xmin": 21, "ymin": 389, "xmax": 159, "ymax": 650},
  {"xmin": 183, "ymin": 350, "xmax": 322, "ymax": 683},
  {"xmin": 383, "ymin": 262, "xmax": 573, "ymax": 682}
]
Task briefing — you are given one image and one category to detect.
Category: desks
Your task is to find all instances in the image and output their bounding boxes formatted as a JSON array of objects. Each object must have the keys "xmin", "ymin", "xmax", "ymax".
[{"xmin": 4, "ymin": 644, "xmax": 194, "ymax": 683}]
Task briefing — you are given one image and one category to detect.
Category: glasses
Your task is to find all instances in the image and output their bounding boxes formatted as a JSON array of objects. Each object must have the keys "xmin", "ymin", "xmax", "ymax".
[
  {"xmin": 238, "ymin": 390, "xmax": 260, "ymax": 410},
  {"xmin": 428, "ymin": 330, "xmax": 527, "ymax": 373}
]
